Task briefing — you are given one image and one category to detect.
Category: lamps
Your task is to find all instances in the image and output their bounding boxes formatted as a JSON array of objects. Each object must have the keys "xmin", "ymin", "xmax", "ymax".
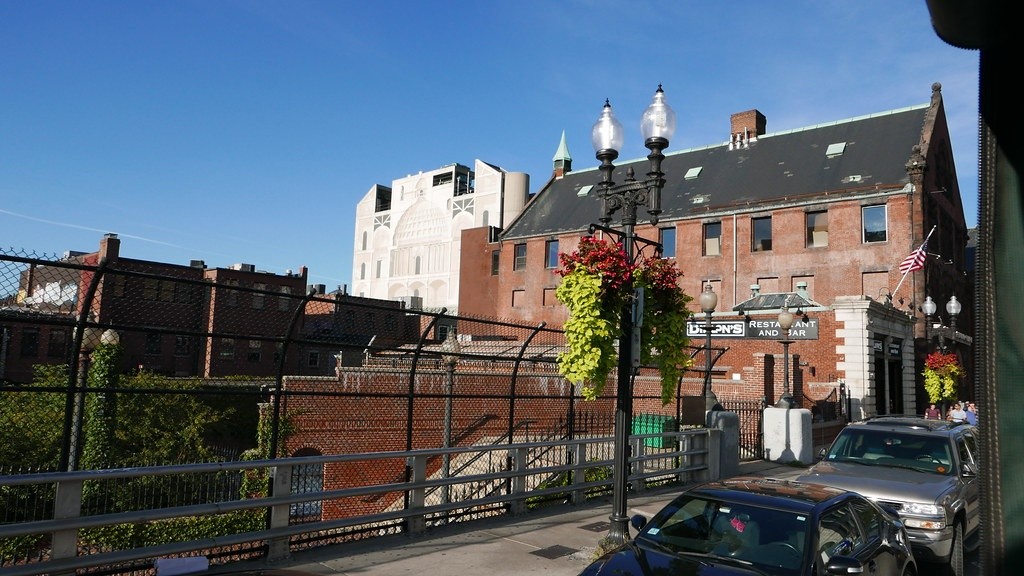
[
  {"xmin": 874, "ymin": 286, "xmax": 923, "ymax": 316},
  {"xmin": 738, "ymin": 309, "xmax": 752, "ymax": 324},
  {"xmin": 795, "ymin": 306, "xmax": 809, "ymax": 324}
]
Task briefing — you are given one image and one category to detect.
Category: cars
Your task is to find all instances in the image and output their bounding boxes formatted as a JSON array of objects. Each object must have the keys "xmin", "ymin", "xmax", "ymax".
[{"xmin": 576, "ymin": 478, "xmax": 918, "ymax": 576}]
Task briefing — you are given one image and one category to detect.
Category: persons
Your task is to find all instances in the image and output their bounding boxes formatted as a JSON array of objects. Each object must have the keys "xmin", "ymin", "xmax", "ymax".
[
  {"xmin": 946, "ymin": 399, "xmax": 979, "ymax": 426},
  {"xmin": 924, "ymin": 403, "xmax": 943, "ymax": 420}
]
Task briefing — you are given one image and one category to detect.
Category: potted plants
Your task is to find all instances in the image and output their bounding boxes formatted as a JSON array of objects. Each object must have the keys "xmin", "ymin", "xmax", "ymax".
[
  {"xmin": 552, "ymin": 235, "xmax": 636, "ymax": 404},
  {"xmin": 627, "ymin": 253, "xmax": 697, "ymax": 408},
  {"xmin": 921, "ymin": 351, "xmax": 967, "ymax": 404}
]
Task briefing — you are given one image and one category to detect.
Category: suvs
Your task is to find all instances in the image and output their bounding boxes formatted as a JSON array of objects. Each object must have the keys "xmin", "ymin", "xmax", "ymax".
[{"xmin": 798, "ymin": 414, "xmax": 982, "ymax": 576}]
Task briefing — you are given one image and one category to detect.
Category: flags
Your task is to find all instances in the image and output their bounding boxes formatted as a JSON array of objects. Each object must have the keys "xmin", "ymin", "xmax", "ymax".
[{"xmin": 899, "ymin": 232, "xmax": 929, "ymax": 274}]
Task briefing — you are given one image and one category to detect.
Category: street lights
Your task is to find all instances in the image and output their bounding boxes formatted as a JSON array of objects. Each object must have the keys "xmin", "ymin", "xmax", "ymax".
[
  {"xmin": 697, "ymin": 279, "xmax": 718, "ymax": 410},
  {"xmin": 922, "ymin": 293, "xmax": 962, "ymax": 421},
  {"xmin": 590, "ymin": 80, "xmax": 673, "ymax": 548},
  {"xmin": 438, "ymin": 329, "xmax": 462, "ymax": 525},
  {"xmin": 776, "ymin": 301, "xmax": 796, "ymax": 409},
  {"xmin": 72, "ymin": 320, "xmax": 96, "ymax": 467}
]
